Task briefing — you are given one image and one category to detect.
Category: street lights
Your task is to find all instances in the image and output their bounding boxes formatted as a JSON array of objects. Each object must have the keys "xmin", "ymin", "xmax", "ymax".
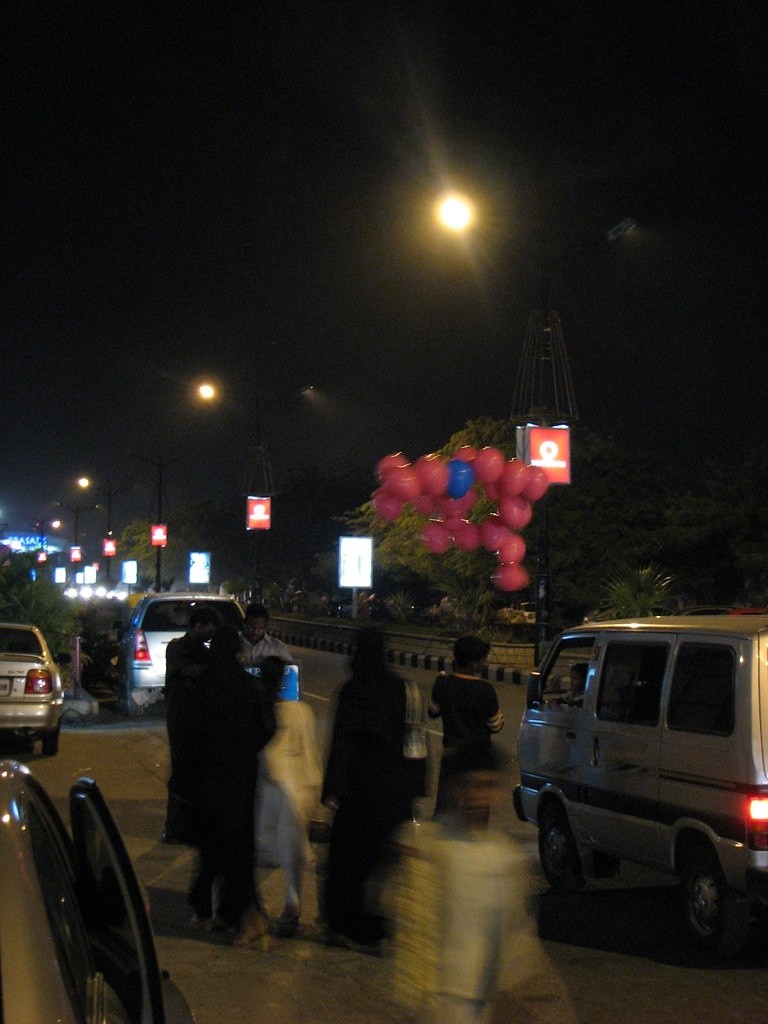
[
  {"xmin": 196, "ymin": 358, "xmax": 266, "ymax": 607},
  {"xmin": 40, "ymin": 516, "xmax": 60, "ymax": 581},
  {"xmin": 124, "ymin": 446, "xmax": 194, "ymax": 592},
  {"xmin": 56, "ymin": 502, "xmax": 99, "ymax": 590},
  {"xmin": 77, "ymin": 468, "xmax": 121, "ymax": 578},
  {"xmin": 428, "ymin": 186, "xmax": 550, "ymax": 667}
]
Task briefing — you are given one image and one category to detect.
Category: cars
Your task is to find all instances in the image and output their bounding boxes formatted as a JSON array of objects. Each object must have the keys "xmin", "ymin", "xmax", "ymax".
[
  {"xmin": 0, "ymin": 624, "xmax": 64, "ymax": 754},
  {"xmin": 0, "ymin": 759, "xmax": 163, "ymax": 1024}
]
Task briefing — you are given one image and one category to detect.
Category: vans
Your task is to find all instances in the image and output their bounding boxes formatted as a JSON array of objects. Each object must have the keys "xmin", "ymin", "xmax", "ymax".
[
  {"xmin": 513, "ymin": 605, "xmax": 768, "ymax": 960},
  {"xmin": 123, "ymin": 592, "xmax": 248, "ymax": 719}
]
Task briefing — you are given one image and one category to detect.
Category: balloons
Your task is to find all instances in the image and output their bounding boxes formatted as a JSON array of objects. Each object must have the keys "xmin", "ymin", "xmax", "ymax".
[{"xmin": 374, "ymin": 445, "xmax": 548, "ymax": 592}]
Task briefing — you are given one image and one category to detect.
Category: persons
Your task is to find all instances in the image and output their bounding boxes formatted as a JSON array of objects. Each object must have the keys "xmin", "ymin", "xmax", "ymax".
[
  {"xmin": 314, "ymin": 630, "xmax": 432, "ymax": 958},
  {"xmin": 236, "ymin": 604, "xmax": 293, "ymax": 664},
  {"xmin": 548, "ymin": 663, "xmax": 589, "ymax": 707},
  {"xmin": 428, "ymin": 636, "xmax": 505, "ymax": 831},
  {"xmin": 395, "ymin": 742, "xmax": 524, "ymax": 1024},
  {"xmin": 166, "ymin": 628, "xmax": 321, "ymax": 948},
  {"xmin": 164, "ymin": 607, "xmax": 224, "ymax": 845}
]
